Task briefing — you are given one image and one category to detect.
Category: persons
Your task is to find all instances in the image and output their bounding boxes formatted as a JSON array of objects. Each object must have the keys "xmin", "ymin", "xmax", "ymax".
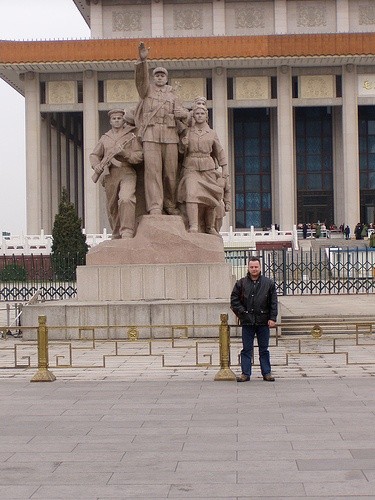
[
  {"xmin": 230, "ymin": 258, "xmax": 278, "ymax": 382},
  {"xmin": 89, "ymin": 108, "xmax": 144, "ymax": 239},
  {"xmin": 178, "ymin": 104, "xmax": 228, "ymax": 235},
  {"xmin": 110, "ymin": 96, "xmax": 210, "ymax": 218},
  {"xmin": 262, "ymin": 218, "xmax": 375, "ymax": 241},
  {"xmin": 134, "ymin": 41, "xmax": 182, "ymax": 215},
  {"xmin": 214, "ymin": 167, "xmax": 232, "ymax": 235}
]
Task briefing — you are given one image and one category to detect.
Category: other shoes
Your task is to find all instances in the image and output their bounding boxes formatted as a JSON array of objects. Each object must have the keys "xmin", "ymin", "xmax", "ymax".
[
  {"xmin": 263, "ymin": 374, "xmax": 274, "ymax": 381},
  {"xmin": 237, "ymin": 374, "xmax": 250, "ymax": 382}
]
